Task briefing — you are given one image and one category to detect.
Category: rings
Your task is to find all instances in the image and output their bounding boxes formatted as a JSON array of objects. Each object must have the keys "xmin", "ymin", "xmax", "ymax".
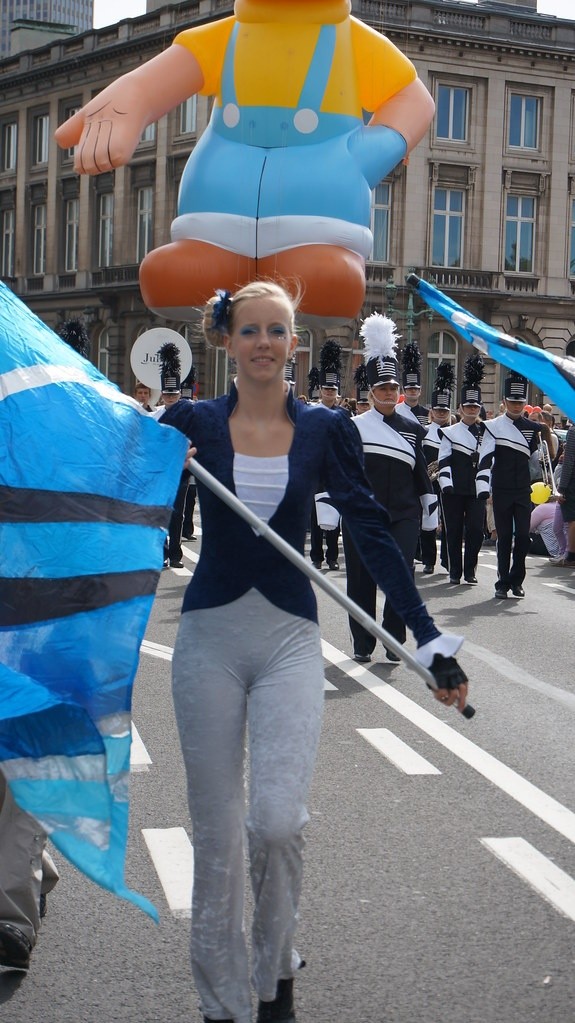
[{"xmin": 442, "ymin": 696, "xmax": 447, "ymax": 701}]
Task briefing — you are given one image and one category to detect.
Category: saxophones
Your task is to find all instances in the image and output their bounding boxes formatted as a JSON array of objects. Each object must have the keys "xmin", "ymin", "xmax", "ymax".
[{"xmin": 426, "ymin": 459, "xmax": 441, "ymax": 481}]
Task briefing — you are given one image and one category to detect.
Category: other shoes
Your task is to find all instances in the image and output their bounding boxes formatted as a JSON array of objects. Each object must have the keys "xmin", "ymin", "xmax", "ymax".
[
  {"xmin": 307, "ymin": 537, "xmax": 574, "ymax": 598},
  {"xmin": 183, "ymin": 534, "xmax": 197, "ymax": 540},
  {"xmin": 163, "ymin": 559, "xmax": 168, "ymax": 567},
  {"xmin": 202, "ymin": 1013, "xmax": 235, "ymax": 1023},
  {"xmin": 355, "ymin": 653, "xmax": 371, "ymax": 662},
  {"xmin": 39, "ymin": 893, "xmax": 46, "ymax": 917},
  {"xmin": 257, "ymin": 959, "xmax": 306, "ymax": 1023},
  {"xmin": 0, "ymin": 922, "xmax": 31, "ymax": 970},
  {"xmin": 169, "ymin": 559, "xmax": 185, "ymax": 568},
  {"xmin": 384, "ymin": 644, "xmax": 401, "ymax": 661}
]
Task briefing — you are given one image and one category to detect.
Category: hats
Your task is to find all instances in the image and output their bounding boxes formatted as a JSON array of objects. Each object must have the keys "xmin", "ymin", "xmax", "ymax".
[
  {"xmin": 360, "ymin": 311, "xmax": 400, "ymax": 392},
  {"xmin": 284, "ymin": 352, "xmax": 296, "ymax": 384},
  {"xmin": 431, "ymin": 361, "xmax": 456, "ymax": 411},
  {"xmin": 180, "ymin": 365, "xmax": 197, "ymax": 400},
  {"xmin": 505, "ymin": 369, "xmax": 528, "ymax": 402},
  {"xmin": 461, "ymin": 354, "xmax": 484, "ymax": 407},
  {"xmin": 59, "ymin": 314, "xmax": 91, "ymax": 357},
  {"xmin": 158, "ymin": 342, "xmax": 181, "ymax": 394},
  {"xmin": 307, "ymin": 366, "xmax": 322, "ymax": 401},
  {"xmin": 400, "ymin": 341, "xmax": 422, "ymax": 388},
  {"xmin": 318, "ymin": 338, "xmax": 342, "ymax": 389},
  {"xmin": 353, "ymin": 364, "xmax": 370, "ymax": 404}
]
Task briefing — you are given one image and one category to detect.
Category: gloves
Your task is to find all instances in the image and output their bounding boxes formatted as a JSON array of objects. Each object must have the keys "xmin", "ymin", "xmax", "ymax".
[
  {"xmin": 445, "ymin": 485, "xmax": 454, "ymax": 495},
  {"xmin": 478, "ymin": 491, "xmax": 490, "ymax": 500}
]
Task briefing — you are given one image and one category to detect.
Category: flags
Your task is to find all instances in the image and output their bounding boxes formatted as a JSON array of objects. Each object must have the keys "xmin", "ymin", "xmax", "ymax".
[
  {"xmin": 413, "ymin": 280, "xmax": 575, "ymax": 425},
  {"xmin": 0, "ymin": 281, "xmax": 190, "ymax": 920}
]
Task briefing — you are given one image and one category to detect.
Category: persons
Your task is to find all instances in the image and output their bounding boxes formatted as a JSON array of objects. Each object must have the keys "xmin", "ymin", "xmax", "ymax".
[
  {"xmin": 475, "ymin": 400, "xmax": 541, "ymax": 598},
  {"xmin": 523, "ymin": 404, "xmax": 575, "ymax": 565},
  {"xmin": 396, "ymin": 388, "xmax": 506, "ymax": 584},
  {"xmin": 299, "ymin": 388, "xmax": 371, "ymax": 570},
  {"xmin": 343, "ymin": 385, "xmax": 439, "ymax": 662},
  {"xmin": 135, "ymin": 384, "xmax": 197, "ymax": 568},
  {"xmin": 158, "ymin": 283, "xmax": 469, "ymax": 1023},
  {"xmin": 0, "ymin": 772, "xmax": 60, "ymax": 969}
]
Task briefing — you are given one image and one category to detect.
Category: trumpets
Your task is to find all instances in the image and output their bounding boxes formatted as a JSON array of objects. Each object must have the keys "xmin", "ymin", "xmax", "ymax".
[{"xmin": 537, "ymin": 431, "xmax": 565, "ymax": 499}]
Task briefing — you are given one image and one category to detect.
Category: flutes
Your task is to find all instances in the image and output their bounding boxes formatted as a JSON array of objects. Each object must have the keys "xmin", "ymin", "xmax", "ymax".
[{"xmin": 471, "ymin": 423, "xmax": 480, "ymax": 483}]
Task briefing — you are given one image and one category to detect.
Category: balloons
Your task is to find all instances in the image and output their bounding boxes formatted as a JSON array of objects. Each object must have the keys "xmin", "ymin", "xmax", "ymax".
[
  {"xmin": 53, "ymin": 0, "xmax": 436, "ymax": 316},
  {"xmin": 530, "ymin": 483, "xmax": 550, "ymax": 504}
]
